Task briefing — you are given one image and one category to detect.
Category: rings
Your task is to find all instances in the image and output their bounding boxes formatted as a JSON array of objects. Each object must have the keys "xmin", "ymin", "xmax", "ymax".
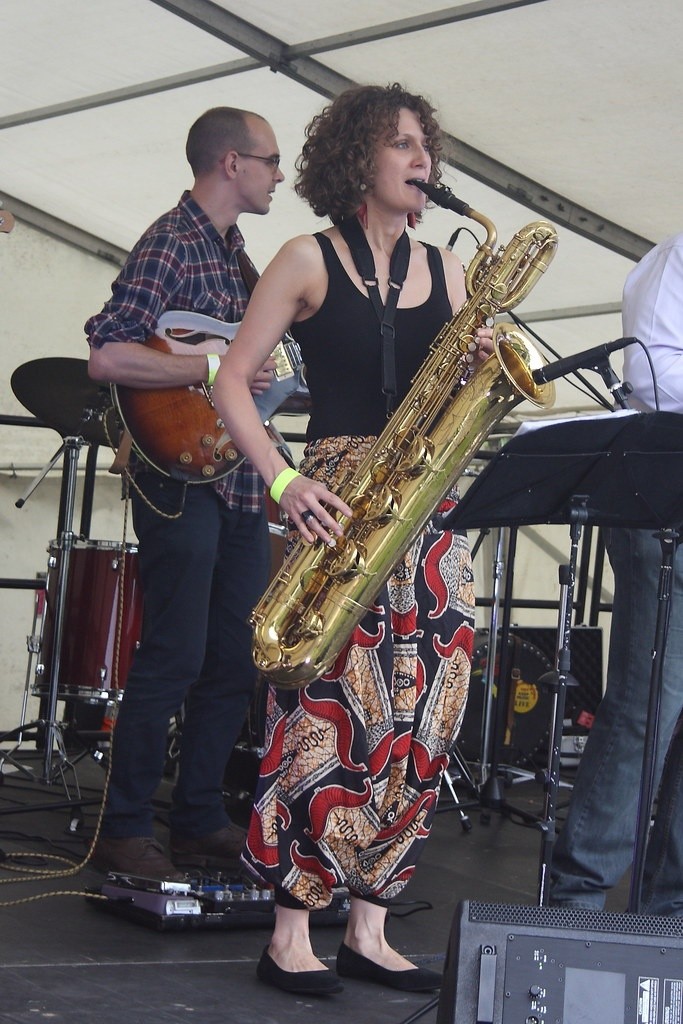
[{"xmin": 302, "ymin": 511, "xmax": 314, "ymax": 524}]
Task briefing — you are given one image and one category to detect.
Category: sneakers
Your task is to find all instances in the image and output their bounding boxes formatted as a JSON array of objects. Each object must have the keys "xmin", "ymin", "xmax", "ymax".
[
  {"xmin": 171, "ymin": 819, "xmax": 250, "ymax": 871},
  {"xmin": 90, "ymin": 833, "xmax": 187, "ymax": 883}
]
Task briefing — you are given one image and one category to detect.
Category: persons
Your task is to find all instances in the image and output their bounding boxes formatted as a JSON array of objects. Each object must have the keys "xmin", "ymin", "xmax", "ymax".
[
  {"xmin": 84, "ymin": 107, "xmax": 283, "ymax": 878},
  {"xmin": 213, "ymin": 82, "xmax": 494, "ymax": 993},
  {"xmin": 546, "ymin": 236, "xmax": 683, "ymax": 919}
]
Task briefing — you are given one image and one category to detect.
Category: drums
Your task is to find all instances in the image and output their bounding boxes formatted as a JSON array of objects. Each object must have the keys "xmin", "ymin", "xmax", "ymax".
[
  {"xmin": 460, "ymin": 631, "xmax": 561, "ymax": 774},
  {"xmin": 263, "ymin": 440, "xmax": 296, "ymax": 576},
  {"xmin": 31, "ymin": 539, "xmax": 144, "ymax": 704}
]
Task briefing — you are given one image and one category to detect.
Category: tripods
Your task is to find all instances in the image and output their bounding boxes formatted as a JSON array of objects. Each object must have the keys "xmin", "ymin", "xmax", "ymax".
[
  {"xmin": 1, "ymin": 400, "xmax": 103, "ymax": 785},
  {"xmin": 428, "ymin": 523, "xmax": 547, "ymax": 831}
]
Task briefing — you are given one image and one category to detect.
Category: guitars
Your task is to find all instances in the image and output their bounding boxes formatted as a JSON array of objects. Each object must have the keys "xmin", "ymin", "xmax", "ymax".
[{"xmin": 110, "ymin": 311, "xmax": 307, "ymax": 500}]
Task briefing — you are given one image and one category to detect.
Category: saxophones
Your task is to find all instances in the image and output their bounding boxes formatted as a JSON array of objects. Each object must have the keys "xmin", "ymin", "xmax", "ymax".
[{"xmin": 247, "ymin": 180, "xmax": 558, "ymax": 689}]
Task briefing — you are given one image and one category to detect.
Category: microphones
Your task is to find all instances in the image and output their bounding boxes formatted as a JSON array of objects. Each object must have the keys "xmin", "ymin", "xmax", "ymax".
[{"xmin": 530, "ymin": 336, "xmax": 638, "ymax": 385}]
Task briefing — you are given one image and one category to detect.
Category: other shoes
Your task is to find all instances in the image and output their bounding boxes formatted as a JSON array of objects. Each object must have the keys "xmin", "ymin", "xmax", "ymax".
[
  {"xmin": 256, "ymin": 946, "xmax": 343, "ymax": 992},
  {"xmin": 335, "ymin": 941, "xmax": 443, "ymax": 991}
]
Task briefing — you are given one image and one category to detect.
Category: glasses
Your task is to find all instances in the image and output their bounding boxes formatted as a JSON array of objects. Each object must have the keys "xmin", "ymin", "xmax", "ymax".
[{"xmin": 219, "ymin": 149, "xmax": 280, "ymax": 174}]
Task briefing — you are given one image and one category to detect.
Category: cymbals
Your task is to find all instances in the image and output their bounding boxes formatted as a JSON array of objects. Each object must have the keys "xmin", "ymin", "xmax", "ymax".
[{"xmin": 10, "ymin": 356, "xmax": 119, "ymax": 449}]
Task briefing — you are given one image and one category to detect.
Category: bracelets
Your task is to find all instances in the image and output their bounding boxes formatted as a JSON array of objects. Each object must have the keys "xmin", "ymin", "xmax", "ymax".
[
  {"xmin": 270, "ymin": 467, "xmax": 302, "ymax": 504},
  {"xmin": 206, "ymin": 355, "xmax": 221, "ymax": 386}
]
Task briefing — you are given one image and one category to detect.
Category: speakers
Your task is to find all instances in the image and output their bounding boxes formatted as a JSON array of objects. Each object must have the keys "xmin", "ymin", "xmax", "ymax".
[{"xmin": 435, "ymin": 899, "xmax": 683, "ymax": 1024}]
